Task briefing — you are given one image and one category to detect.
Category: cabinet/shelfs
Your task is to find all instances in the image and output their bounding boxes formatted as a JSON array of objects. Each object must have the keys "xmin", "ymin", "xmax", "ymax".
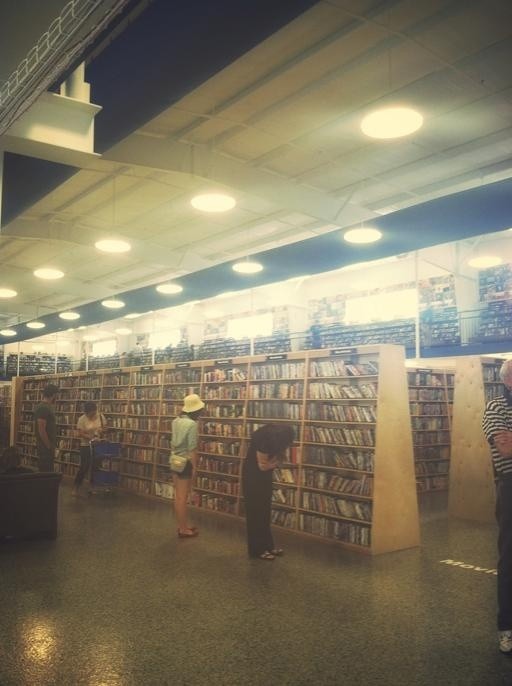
[{"xmin": 0, "ymin": 306, "xmax": 512, "ymax": 557}]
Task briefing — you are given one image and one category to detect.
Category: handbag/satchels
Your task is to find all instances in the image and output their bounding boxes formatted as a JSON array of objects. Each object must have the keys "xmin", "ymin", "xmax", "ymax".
[{"xmin": 168, "ymin": 454, "xmax": 188, "ymax": 474}]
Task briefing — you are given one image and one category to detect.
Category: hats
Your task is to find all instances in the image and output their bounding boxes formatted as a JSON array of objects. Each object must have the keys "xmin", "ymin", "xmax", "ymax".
[{"xmin": 181, "ymin": 394, "xmax": 205, "ymax": 412}]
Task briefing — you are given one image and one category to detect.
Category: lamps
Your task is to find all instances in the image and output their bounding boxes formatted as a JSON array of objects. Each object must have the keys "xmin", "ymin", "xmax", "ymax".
[
  {"xmin": 342, "ymin": 219, "xmax": 382, "ymax": 244},
  {"xmin": 1, "ymin": 205, "xmax": 265, "ymax": 309},
  {"xmin": 189, "ymin": 105, "xmax": 238, "ymax": 213},
  {"xmin": 362, "ymin": 1, "xmax": 424, "ymax": 142}
]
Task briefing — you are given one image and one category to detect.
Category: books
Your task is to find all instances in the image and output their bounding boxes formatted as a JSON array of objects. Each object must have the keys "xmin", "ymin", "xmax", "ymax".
[
  {"xmin": 243, "ymin": 356, "xmax": 377, "ymax": 546},
  {"xmin": 408, "ymin": 373, "xmax": 454, "ymax": 491},
  {"xmin": 0, "ymin": 385, "xmax": 12, "ymax": 449},
  {"xmin": 15, "ymin": 379, "xmax": 58, "ymax": 465},
  {"xmin": 78, "ymin": 349, "xmax": 152, "ymax": 372},
  {"xmin": 154, "ymin": 338, "xmax": 250, "ymax": 362},
  {"xmin": 483, "ymin": 365, "xmax": 505, "ymax": 404},
  {"xmin": 303, "ymin": 318, "xmax": 416, "ymax": 359},
  {"xmin": 418, "ymin": 263, "xmax": 512, "ymax": 342},
  {"xmin": 53, "ymin": 375, "xmax": 101, "ymax": 474},
  {"xmin": 0, "ymin": 351, "xmax": 72, "ymax": 381},
  {"xmin": 193, "ymin": 368, "xmax": 246, "ymax": 516},
  {"xmin": 104, "ymin": 367, "xmax": 201, "ymax": 502},
  {"xmin": 254, "ymin": 333, "xmax": 291, "ymax": 355}
]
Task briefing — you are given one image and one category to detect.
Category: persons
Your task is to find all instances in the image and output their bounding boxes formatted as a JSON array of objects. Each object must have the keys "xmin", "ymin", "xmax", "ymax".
[
  {"xmin": 242, "ymin": 420, "xmax": 296, "ymax": 561},
  {"xmin": 481, "ymin": 360, "xmax": 511, "ymax": 656},
  {"xmin": 133, "ymin": 342, "xmax": 143, "ymax": 366},
  {"xmin": 33, "ymin": 384, "xmax": 59, "ymax": 473},
  {"xmin": 1, "ymin": 447, "xmax": 28, "ymax": 474},
  {"xmin": 72, "ymin": 402, "xmax": 107, "ymax": 496},
  {"xmin": 169, "ymin": 394, "xmax": 206, "ymax": 537}
]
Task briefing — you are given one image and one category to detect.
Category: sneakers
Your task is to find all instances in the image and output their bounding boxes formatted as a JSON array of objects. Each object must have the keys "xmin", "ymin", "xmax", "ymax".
[{"xmin": 497, "ymin": 629, "xmax": 512, "ymax": 654}]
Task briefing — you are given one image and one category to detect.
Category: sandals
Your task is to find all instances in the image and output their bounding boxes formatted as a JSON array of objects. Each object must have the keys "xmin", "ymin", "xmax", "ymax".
[
  {"xmin": 259, "ymin": 547, "xmax": 285, "ymax": 560},
  {"xmin": 176, "ymin": 526, "xmax": 200, "ymax": 539}
]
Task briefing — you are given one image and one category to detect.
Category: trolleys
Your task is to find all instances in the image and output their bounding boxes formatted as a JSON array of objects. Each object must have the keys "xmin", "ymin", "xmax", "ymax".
[{"xmin": 88, "ymin": 438, "xmax": 122, "ymax": 499}]
